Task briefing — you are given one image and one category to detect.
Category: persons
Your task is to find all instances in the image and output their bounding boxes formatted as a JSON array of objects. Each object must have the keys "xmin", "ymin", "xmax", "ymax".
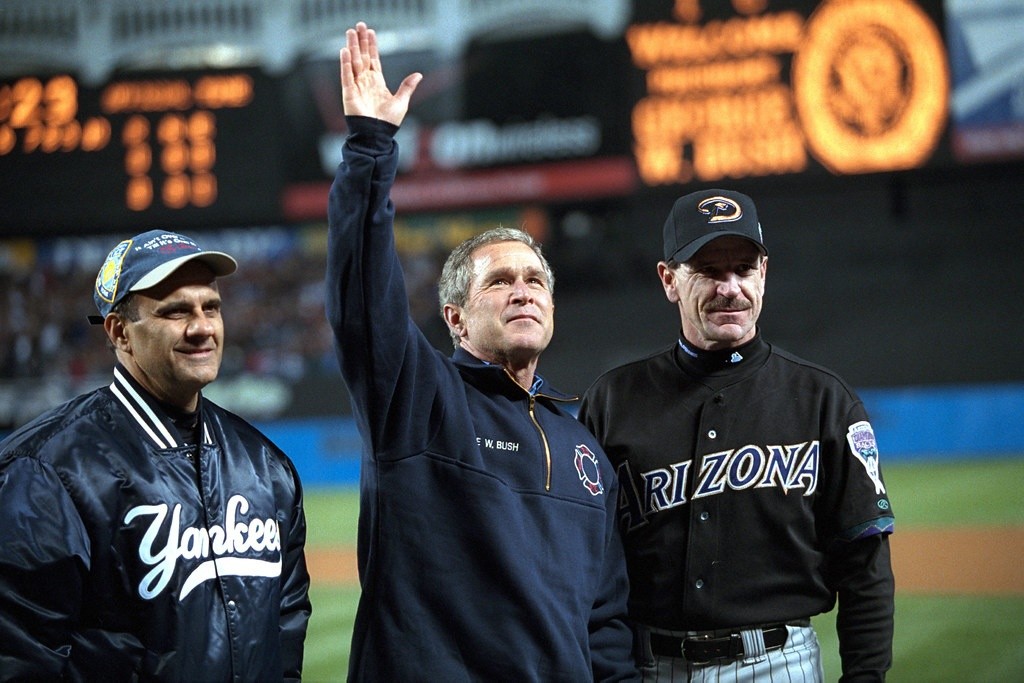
[
  {"xmin": 325, "ymin": 21, "xmax": 642, "ymax": 683},
  {"xmin": 2, "ymin": 232, "xmax": 312, "ymax": 683},
  {"xmin": 574, "ymin": 189, "xmax": 898, "ymax": 683}
]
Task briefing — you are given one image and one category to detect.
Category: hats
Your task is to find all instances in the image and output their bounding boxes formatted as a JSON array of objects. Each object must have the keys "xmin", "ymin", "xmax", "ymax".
[
  {"xmin": 88, "ymin": 225, "xmax": 240, "ymax": 328},
  {"xmin": 661, "ymin": 186, "xmax": 771, "ymax": 270}
]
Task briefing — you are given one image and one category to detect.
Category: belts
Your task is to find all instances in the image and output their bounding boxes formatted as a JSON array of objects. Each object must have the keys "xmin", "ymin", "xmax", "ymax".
[{"xmin": 627, "ymin": 625, "xmax": 787, "ymax": 664}]
